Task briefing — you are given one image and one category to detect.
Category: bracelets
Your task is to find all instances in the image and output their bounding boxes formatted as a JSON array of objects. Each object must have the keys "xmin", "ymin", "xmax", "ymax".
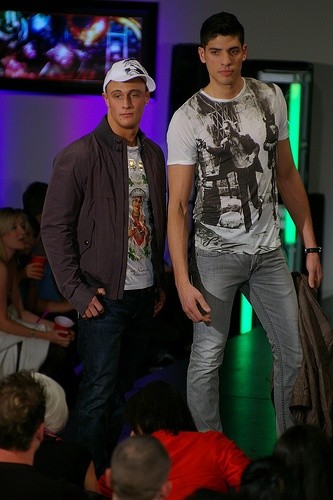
[{"xmin": 304, "ymin": 246, "xmax": 323, "ymax": 254}]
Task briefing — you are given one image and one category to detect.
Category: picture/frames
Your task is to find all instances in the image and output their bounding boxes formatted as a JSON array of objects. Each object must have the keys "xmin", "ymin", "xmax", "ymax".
[{"xmin": 0, "ymin": 0, "xmax": 159, "ymax": 98}]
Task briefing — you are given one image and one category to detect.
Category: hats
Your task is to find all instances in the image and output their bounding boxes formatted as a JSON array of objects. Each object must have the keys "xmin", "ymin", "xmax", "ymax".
[
  {"xmin": 125, "ymin": 369, "xmax": 173, "ymax": 400},
  {"xmin": 103, "ymin": 58, "xmax": 156, "ymax": 93}
]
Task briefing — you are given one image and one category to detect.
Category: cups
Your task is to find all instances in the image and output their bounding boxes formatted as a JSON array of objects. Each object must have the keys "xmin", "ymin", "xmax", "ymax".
[
  {"xmin": 54, "ymin": 316, "xmax": 74, "ymax": 338},
  {"xmin": 32, "ymin": 256, "xmax": 46, "ymax": 273}
]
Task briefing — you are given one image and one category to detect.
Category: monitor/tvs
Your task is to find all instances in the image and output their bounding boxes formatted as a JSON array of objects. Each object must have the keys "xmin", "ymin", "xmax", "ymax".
[{"xmin": 0, "ymin": 1, "xmax": 160, "ymax": 98}]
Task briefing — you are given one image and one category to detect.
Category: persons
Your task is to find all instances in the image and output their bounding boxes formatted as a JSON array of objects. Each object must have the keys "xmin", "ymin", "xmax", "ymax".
[
  {"xmin": 20, "ymin": 180, "xmax": 87, "ymax": 395},
  {"xmin": 0, "ymin": 207, "xmax": 75, "ymax": 378},
  {"xmin": 20, "ymin": 371, "xmax": 88, "ymax": 499},
  {"xmin": 105, "ymin": 435, "xmax": 173, "ymax": 500},
  {"xmin": 0, "ymin": 374, "xmax": 102, "ymax": 500},
  {"xmin": 239, "ymin": 455, "xmax": 291, "ymax": 500},
  {"xmin": 39, "ymin": 58, "xmax": 169, "ymax": 460},
  {"xmin": 165, "ymin": 12, "xmax": 323, "ymax": 437},
  {"xmin": 272, "ymin": 422, "xmax": 333, "ymax": 500},
  {"xmin": 94, "ymin": 381, "xmax": 253, "ymax": 500}
]
